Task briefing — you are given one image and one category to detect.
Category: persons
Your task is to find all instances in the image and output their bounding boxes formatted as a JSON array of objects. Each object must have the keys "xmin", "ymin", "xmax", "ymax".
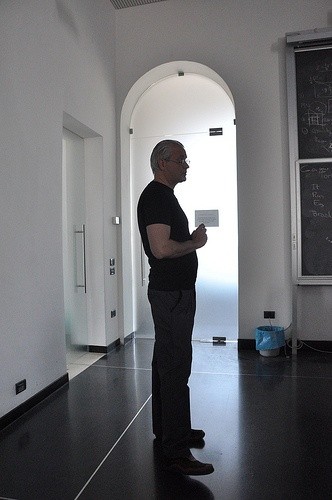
[{"xmin": 136, "ymin": 140, "xmax": 215, "ymax": 476}]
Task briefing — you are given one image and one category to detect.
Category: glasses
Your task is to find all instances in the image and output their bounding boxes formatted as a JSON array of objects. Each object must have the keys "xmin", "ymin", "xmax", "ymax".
[{"xmin": 165, "ymin": 158, "xmax": 190, "ymax": 166}]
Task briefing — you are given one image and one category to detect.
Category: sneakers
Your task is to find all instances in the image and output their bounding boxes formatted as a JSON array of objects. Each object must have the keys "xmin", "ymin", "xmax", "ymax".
[
  {"xmin": 155, "ymin": 447, "xmax": 214, "ymax": 476},
  {"xmin": 155, "ymin": 428, "xmax": 205, "ymax": 440}
]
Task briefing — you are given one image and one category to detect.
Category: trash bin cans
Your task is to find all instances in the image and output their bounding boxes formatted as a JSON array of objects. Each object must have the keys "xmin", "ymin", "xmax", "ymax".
[{"xmin": 256, "ymin": 325, "xmax": 285, "ymax": 358}]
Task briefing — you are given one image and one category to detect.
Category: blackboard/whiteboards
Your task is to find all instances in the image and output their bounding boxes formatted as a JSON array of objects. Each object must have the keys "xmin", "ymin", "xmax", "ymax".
[{"xmin": 285, "ymin": 26, "xmax": 332, "ymax": 286}]
